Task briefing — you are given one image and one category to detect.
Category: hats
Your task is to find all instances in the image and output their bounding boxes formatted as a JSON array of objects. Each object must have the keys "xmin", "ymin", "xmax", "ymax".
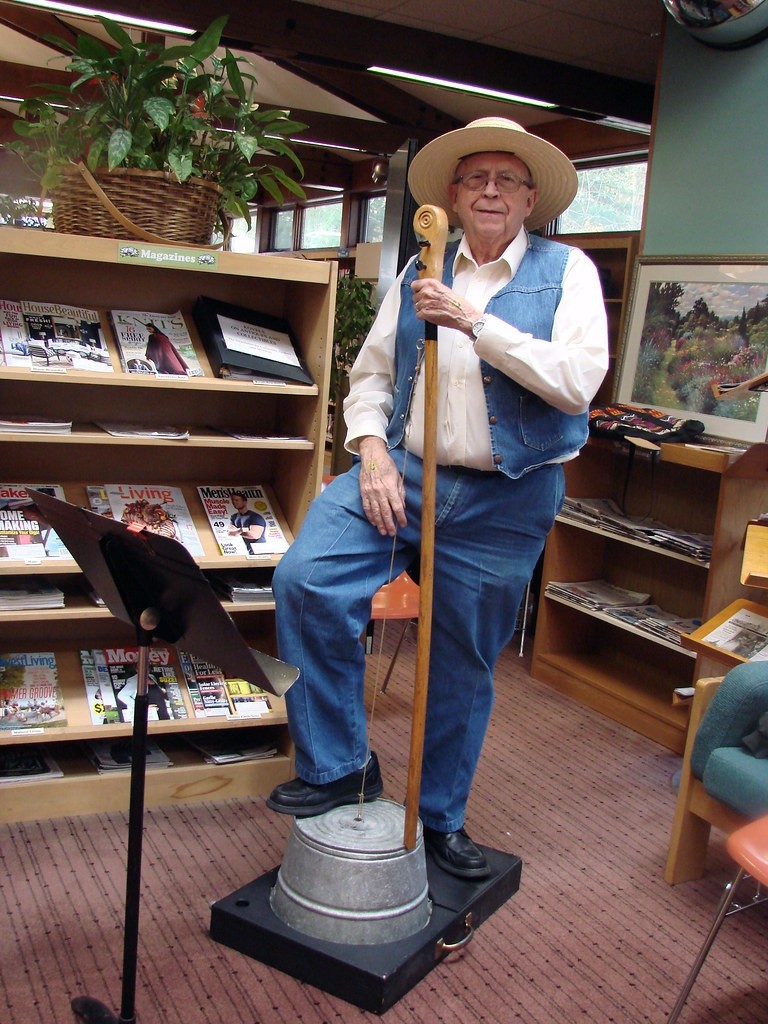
[{"xmin": 408, "ymin": 116, "xmax": 578, "ymax": 233}]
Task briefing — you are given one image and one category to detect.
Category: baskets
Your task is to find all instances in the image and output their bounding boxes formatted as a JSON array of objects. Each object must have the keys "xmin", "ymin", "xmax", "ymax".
[{"xmin": 52, "ymin": 161, "xmax": 235, "ymax": 249}]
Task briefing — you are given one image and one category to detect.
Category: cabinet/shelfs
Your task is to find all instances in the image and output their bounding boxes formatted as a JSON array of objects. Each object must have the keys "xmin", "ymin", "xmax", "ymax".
[
  {"xmin": 551, "ymin": 236, "xmax": 638, "ymax": 405},
  {"xmin": 325, "ymin": 242, "xmax": 383, "ymax": 478},
  {"xmin": 0, "ymin": 225, "xmax": 339, "ymax": 825},
  {"xmin": 531, "ymin": 441, "xmax": 767, "ymax": 756}
]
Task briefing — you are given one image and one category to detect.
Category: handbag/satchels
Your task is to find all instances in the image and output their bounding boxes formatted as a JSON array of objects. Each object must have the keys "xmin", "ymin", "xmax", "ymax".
[{"xmin": 589, "ymin": 402, "xmax": 705, "ymax": 522}]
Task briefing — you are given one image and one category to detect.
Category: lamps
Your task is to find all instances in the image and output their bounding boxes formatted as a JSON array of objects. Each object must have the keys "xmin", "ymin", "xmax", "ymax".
[{"xmin": 371, "ymin": 152, "xmax": 390, "ymax": 186}]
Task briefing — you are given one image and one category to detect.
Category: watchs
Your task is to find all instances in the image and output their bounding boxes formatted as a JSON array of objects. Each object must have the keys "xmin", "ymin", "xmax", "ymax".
[{"xmin": 469, "ymin": 313, "xmax": 488, "ymax": 342}]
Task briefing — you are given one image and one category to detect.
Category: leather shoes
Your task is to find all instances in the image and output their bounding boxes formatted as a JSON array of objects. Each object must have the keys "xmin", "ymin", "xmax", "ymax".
[
  {"xmin": 266, "ymin": 750, "xmax": 382, "ymax": 816},
  {"xmin": 424, "ymin": 824, "xmax": 491, "ymax": 877}
]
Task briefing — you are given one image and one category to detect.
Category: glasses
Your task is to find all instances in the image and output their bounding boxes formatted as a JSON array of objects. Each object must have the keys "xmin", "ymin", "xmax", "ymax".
[{"xmin": 455, "ymin": 171, "xmax": 531, "ymax": 193}]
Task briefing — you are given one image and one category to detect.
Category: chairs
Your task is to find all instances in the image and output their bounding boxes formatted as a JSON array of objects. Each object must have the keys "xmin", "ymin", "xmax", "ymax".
[
  {"xmin": 321, "ymin": 475, "xmax": 420, "ymax": 695},
  {"xmin": 666, "ymin": 814, "xmax": 768, "ymax": 1024},
  {"xmin": 665, "ymin": 661, "xmax": 768, "ymax": 886}
]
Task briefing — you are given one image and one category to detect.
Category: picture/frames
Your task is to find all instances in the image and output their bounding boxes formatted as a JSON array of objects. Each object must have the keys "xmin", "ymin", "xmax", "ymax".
[{"xmin": 611, "ymin": 254, "xmax": 767, "ymax": 450}]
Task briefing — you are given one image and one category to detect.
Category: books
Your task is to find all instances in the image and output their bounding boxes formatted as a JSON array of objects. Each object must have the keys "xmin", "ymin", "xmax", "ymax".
[
  {"xmin": 0, "ymin": 580, "xmax": 273, "ymax": 609},
  {"xmin": 0, "ymin": 299, "xmax": 205, "ymax": 377},
  {"xmin": 0, "ymin": 739, "xmax": 277, "ymax": 787},
  {"xmin": 0, "ymin": 643, "xmax": 272, "ymax": 731},
  {"xmin": 0, "ymin": 415, "xmax": 307, "ymax": 440},
  {"xmin": 0, "ymin": 481, "xmax": 291, "ymax": 557}
]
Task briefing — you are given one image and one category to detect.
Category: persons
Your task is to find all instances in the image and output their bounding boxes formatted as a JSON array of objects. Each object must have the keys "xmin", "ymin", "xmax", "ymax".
[
  {"xmin": 117, "ymin": 664, "xmax": 169, "ymax": 718},
  {"xmin": 145, "ymin": 323, "xmax": 188, "ymax": 375},
  {"xmin": 264, "ymin": 117, "xmax": 610, "ymax": 877},
  {"xmin": 228, "ymin": 493, "xmax": 266, "ymax": 555}
]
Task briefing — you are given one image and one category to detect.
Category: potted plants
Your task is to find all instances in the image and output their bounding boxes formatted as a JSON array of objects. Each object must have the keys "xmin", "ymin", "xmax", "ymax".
[{"xmin": 0, "ymin": 15, "xmax": 311, "ymax": 250}]
